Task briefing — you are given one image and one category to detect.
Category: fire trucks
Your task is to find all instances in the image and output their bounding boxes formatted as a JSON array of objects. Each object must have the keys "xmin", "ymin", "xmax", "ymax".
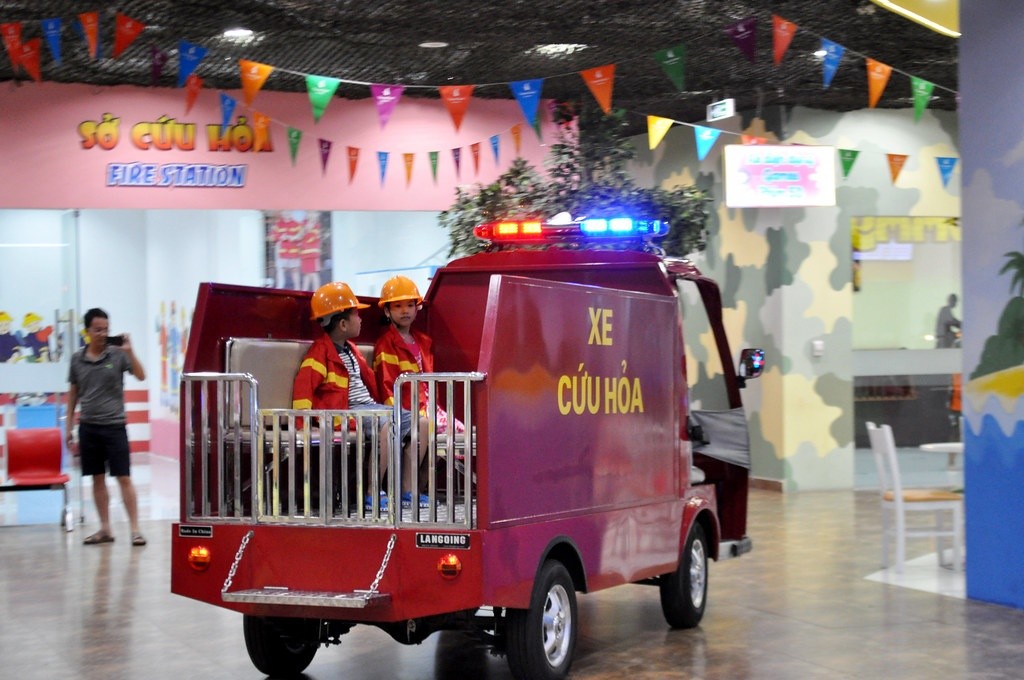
[{"xmin": 162, "ymin": 217, "xmax": 768, "ymax": 680}]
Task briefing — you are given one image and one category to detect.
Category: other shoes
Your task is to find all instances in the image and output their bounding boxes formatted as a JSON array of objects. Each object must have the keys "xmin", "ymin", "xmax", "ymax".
[
  {"xmin": 399, "ymin": 491, "xmax": 441, "ymax": 507},
  {"xmin": 365, "ymin": 492, "xmax": 396, "ymax": 511}
]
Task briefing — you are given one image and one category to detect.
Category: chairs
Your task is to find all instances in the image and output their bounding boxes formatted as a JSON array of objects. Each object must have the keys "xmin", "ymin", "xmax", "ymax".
[
  {"xmin": 865, "ymin": 420, "xmax": 964, "ymax": 576},
  {"xmin": 3, "ymin": 428, "xmax": 74, "ymax": 534}
]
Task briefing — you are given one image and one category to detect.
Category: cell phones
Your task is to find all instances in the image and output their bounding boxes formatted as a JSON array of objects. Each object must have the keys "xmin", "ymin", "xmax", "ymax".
[{"xmin": 106, "ymin": 336, "xmax": 123, "ymax": 346}]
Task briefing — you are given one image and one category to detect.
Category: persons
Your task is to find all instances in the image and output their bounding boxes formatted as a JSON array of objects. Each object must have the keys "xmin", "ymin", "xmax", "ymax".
[
  {"xmin": 64, "ymin": 308, "xmax": 146, "ymax": 546},
  {"xmin": 292, "ymin": 282, "xmax": 439, "ymax": 512},
  {"xmin": 372, "ymin": 275, "xmax": 477, "ymax": 486}
]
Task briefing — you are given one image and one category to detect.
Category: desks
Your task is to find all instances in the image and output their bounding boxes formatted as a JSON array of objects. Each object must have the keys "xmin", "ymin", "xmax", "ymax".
[{"xmin": 918, "ymin": 441, "xmax": 965, "ymax": 454}]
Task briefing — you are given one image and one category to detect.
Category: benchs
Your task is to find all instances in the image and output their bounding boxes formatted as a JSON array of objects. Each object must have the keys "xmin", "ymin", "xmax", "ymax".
[{"xmin": 222, "ymin": 336, "xmax": 476, "ymax": 520}]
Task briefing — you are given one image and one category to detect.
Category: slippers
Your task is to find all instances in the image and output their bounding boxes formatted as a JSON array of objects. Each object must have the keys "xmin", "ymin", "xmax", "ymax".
[
  {"xmin": 131, "ymin": 532, "xmax": 146, "ymax": 545},
  {"xmin": 83, "ymin": 530, "xmax": 114, "ymax": 543}
]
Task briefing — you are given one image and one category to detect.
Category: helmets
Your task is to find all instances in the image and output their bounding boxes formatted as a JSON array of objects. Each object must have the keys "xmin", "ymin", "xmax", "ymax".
[
  {"xmin": 308, "ymin": 282, "xmax": 370, "ymax": 321},
  {"xmin": 378, "ymin": 275, "xmax": 423, "ymax": 307}
]
